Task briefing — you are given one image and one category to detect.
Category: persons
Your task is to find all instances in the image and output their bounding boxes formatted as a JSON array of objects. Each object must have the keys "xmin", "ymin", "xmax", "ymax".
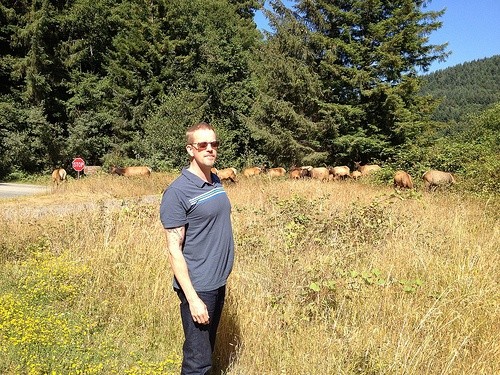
[{"xmin": 160, "ymin": 123, "xmax": 235, "ymax": 375}]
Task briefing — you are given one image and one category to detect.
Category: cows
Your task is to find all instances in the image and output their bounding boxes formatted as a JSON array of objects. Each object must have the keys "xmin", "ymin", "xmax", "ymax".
[
  {"xmin": 110, "ymin": 166, "xmax": 150, "ymax": 177},
  {"xmin": 422, "ymin": 170, "xmax": 456, "ymax": 190},
  {"xmin": 52, "ymin": 168, "xmax": 66, "ymax": 186},
  {"xmin": 212, "ymin": 160, "xmax": 381, "ymax": 183},
  {"xmin": 394, "ymin": 172, "xmax": 415, "ymax": 190}
]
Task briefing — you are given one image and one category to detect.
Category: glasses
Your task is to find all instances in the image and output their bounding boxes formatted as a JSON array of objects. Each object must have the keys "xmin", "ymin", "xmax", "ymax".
[{"xmin": 189, "ymin": 141, "xmax": 219, "ymax": 150}]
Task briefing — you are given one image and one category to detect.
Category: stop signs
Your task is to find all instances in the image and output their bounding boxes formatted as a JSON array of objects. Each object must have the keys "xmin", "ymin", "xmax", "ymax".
[{"xmin": 72, "ymin": 158, "xmax": 85, "ymax": 172}]
